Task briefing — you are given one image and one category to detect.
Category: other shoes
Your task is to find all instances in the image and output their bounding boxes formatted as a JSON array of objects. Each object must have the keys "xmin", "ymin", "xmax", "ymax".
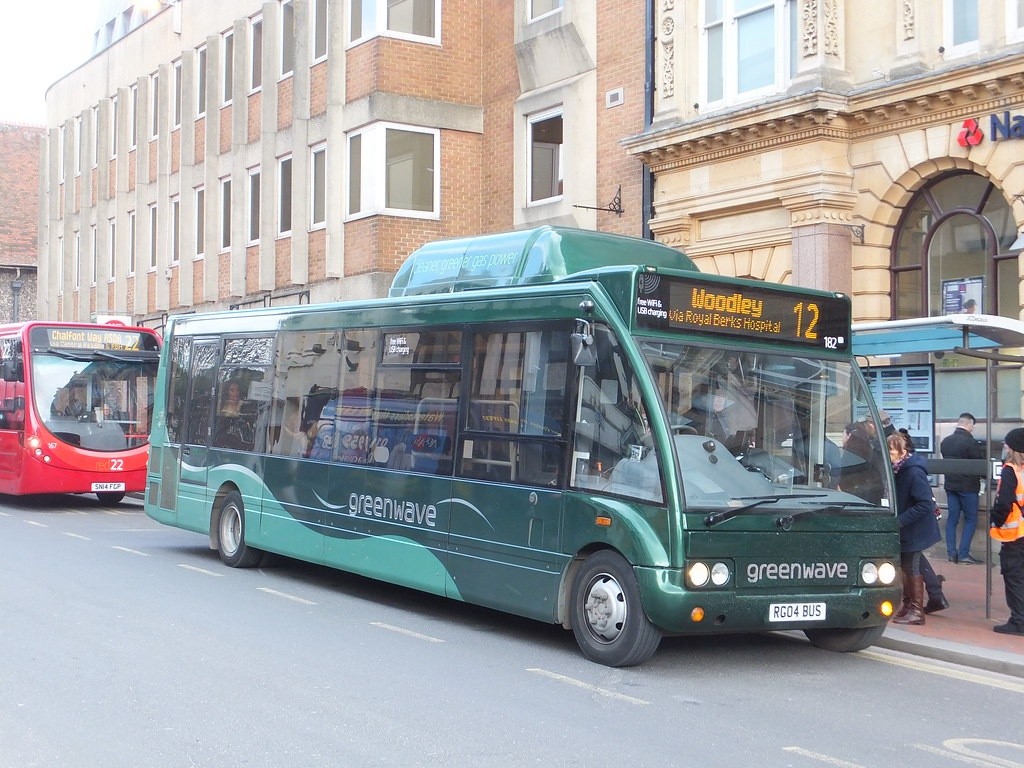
[
  {"xmin": 994, "ymin": 622, "xmax": 1024, "ymax": 635},
  {"xmin": 957, "ymin": 554, "xmax": 984, "ymax": 565},
  {"xmin": 948, "ymin": 556, "xmax": 958, "ymax": 564}
]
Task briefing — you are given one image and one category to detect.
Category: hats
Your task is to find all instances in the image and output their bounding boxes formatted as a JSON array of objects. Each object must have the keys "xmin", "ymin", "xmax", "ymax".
[
  {"xmin": 1004, "ymin": 428, "xmax": 1024, "ymax": 453},
  {"xmin": 857, "ymin": 407, "xmax": 893, "ymax": 422}
]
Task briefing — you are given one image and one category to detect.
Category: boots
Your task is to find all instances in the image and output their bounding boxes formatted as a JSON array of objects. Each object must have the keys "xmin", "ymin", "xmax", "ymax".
[{"xmin": 893, "ymin": 573, "xmax": 925, "ymax": 624}]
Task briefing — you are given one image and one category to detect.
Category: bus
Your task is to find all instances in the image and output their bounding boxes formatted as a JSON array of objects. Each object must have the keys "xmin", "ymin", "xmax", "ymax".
[
  {"xmin": 0, "ymin": 320, "xmax": 163, "ymax": 507},
  {"xmin": 308, "ymin": 396, "xmax": 499, "ymax": 482},
  {"xmin": 145, "ymin": 223, "xmax": 904, "ymax": 668}
]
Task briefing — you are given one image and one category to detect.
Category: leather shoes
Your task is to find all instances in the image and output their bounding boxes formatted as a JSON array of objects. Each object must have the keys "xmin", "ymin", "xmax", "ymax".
[{"xmin": 923, "ymin": 593, "xmax": 949, "ymax": 614}]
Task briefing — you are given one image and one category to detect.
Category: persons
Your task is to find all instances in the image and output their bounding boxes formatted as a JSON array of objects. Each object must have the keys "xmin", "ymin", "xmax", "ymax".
[
  {"xmin": 680, "ymin": 384, "xmax": 738, "ymax": 457},
  {"xmin": 840, "ymin": 408, "xmax": 949, "ymax": 625},
  {"xmin": 989, "ymin": 427, "xmax": 1024, "ymax": 636},
  {"xmin": 940, "ymin": 413, "xmax": 986, "ymax": 564},
  {"xmin": 222, "ymin": 379, "xmax": 241, "ymax": 405},
  {"xmin": 963, "ymin": 298, "xmax": 977, "ymax": 314},
  {"xmin": 797, "ymin": 433, "xmax": 840, "ymax": 489},
  {"xmin": 104, "ymin": 404, "xmax": 112, "ymax": 420}
]
